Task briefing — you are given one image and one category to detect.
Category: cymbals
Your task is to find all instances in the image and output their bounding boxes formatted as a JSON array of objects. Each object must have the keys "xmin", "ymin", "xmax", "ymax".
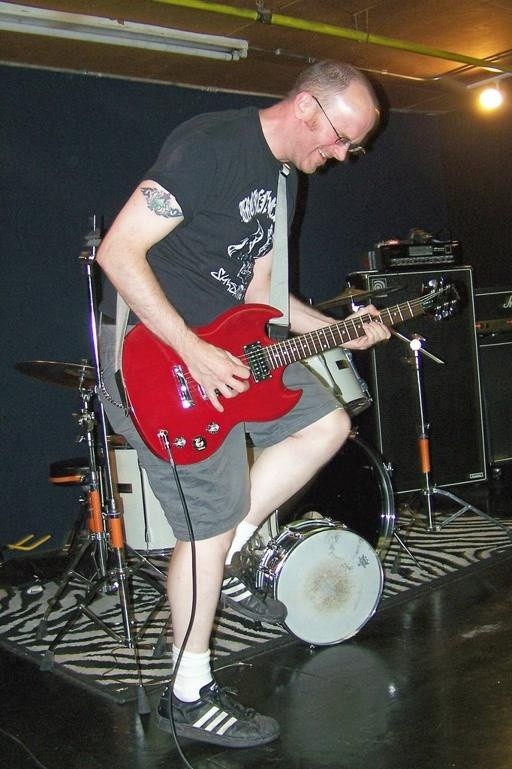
[
  {"xmin": 312, "ymin": 283, "xmax": 405, "ymax": 310},
  {"xmin": 12, "ymin": 359, "xmax": 97, "ymax": 389}
]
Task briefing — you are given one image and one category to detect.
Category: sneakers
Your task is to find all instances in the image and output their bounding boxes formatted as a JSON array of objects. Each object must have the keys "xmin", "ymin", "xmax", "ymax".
[
  {"xmin": 155, "ymin": 675, "xmax": 281, "ymax": 747},
  {"xmin": 220, "ymin": 553, "xmax": 286, "ymax": 626}
]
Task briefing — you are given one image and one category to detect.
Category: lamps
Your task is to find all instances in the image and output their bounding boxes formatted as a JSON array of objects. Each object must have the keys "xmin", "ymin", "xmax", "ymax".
[{"xmin": 0, "ymin": 0, "xmax": 248, "ymax": 62}]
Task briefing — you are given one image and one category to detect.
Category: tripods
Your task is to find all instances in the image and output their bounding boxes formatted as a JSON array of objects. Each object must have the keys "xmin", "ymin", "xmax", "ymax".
[
  {"xmin": 393, "ymin": 328, "xmax": 512, "ymax": 541},
  {"xmin": 39, "ymin": 274, "xmax": 163, "ymax": 720},
  {"xmin": 34, "ymin": 401, "xmax": 162, "ymax": 641}
]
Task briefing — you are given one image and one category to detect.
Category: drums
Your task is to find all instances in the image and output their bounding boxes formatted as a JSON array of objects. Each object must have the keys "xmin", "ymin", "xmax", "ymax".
[
  {"xmin": 300, "ymin": 346, "xmax": 373, "ymax": 417},
  {"xmin": 255, "ymin": 518, "xmax": 386, "ymax": 646},
  {"xmin": 276, "ymin": 430, "xmax": 396, "ymax": 563},
  {"xmin": 112, "ymin": 445, "xmax": 178, "ymax": 557}
]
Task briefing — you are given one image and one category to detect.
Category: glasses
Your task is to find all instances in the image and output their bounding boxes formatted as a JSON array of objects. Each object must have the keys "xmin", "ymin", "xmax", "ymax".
[{"xmin": 310, "ymin": 96, "xmax": 362, "ymax": 154}]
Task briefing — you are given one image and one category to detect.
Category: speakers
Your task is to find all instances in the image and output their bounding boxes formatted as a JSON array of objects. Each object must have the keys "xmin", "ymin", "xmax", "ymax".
[
  {"xmin": 473, "ymin": 317, "xmax": 512, "ymax": 463},
  {"xmin": 348, "ymin": 265, "xmax": 487, "ymax": 495}
]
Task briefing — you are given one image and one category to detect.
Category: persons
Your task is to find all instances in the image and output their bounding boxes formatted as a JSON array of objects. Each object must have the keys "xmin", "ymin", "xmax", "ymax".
[{"xmin": 87, "ymin": 56, "xmax": 394, "ymax": 752}]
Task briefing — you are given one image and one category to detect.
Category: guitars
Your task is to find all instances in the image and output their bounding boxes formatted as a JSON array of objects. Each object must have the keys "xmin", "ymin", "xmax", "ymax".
[{"xmin": 113, "ymin": 278, "xmax": 469, "ymax": 465}]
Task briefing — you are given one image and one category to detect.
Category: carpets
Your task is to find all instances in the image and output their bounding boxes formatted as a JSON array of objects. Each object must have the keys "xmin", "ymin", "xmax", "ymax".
[{"xmin": 1, "ymin": 507, "xmax": 511, "ymax": 709}]
